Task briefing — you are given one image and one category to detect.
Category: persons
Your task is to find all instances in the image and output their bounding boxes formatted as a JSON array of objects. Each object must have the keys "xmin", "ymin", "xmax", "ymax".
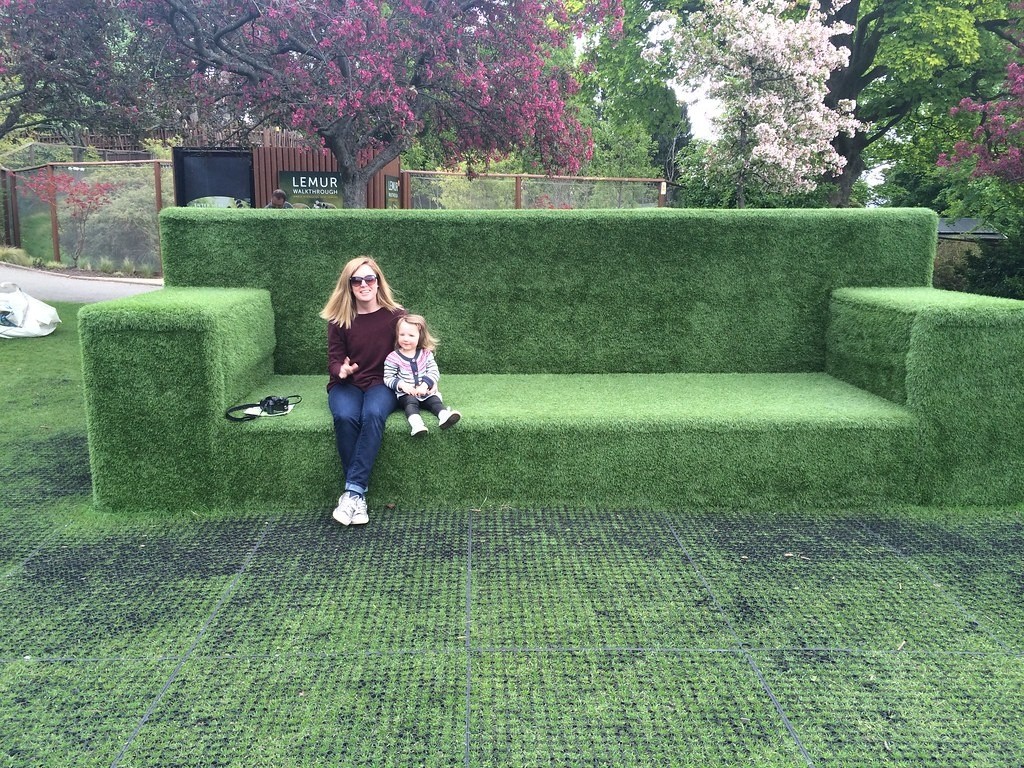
[
  {"xmin": 318, "ymin": 255, "xmax": 409, "ymax": 526},
  {"xmin": 383, "ymin": 313, "xmax": 462, "ymax": 436},
  {"xmin": 263, "ymin": 189, "xmax": 294, "ymax": 209}
]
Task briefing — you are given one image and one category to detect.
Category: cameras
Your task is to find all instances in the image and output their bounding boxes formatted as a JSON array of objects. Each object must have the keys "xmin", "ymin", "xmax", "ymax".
[{"xmin": 260, "ymin": 395, "xmax": 289, "ymax": 414}]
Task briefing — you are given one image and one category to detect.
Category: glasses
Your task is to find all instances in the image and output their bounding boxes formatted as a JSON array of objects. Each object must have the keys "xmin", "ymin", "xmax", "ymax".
[
  {"xmin": 271, "ymin": 198, "xmax": 283, "ymax": 208},
  {"xmin": 349, "ymin": 275, "xmax": 378, "ymax": 287}
]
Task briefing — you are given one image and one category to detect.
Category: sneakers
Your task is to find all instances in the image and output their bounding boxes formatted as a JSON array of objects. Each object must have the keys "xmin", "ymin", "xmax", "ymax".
[
  {"xmin": 333, "ymin": 492, "xmax": 359, "ymax": 526},
  {"xmin": 351, "ymin": 496, "xmax": 369, "ymax": 525}
]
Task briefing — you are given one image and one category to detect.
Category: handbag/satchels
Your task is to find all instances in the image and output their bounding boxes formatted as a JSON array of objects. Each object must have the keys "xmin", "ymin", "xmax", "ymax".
[{"xmin": 226, "ymin": 394, "xmax": 302, "ymax": 422}]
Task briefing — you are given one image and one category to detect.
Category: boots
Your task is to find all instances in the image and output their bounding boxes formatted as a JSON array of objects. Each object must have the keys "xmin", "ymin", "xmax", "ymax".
[
  {"xmin": 438, "ymin": 406, "xmax": 461, "ymax": 429},
  {"xmin": 408, "ymin": 414, "xmax": 428, "ymax": 436}
]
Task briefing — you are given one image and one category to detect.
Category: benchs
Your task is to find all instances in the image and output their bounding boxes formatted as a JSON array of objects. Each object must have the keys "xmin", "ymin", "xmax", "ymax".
[{"xmin": 76, "ymin": 209, "xmax": 1024, "ymax": 510}]
